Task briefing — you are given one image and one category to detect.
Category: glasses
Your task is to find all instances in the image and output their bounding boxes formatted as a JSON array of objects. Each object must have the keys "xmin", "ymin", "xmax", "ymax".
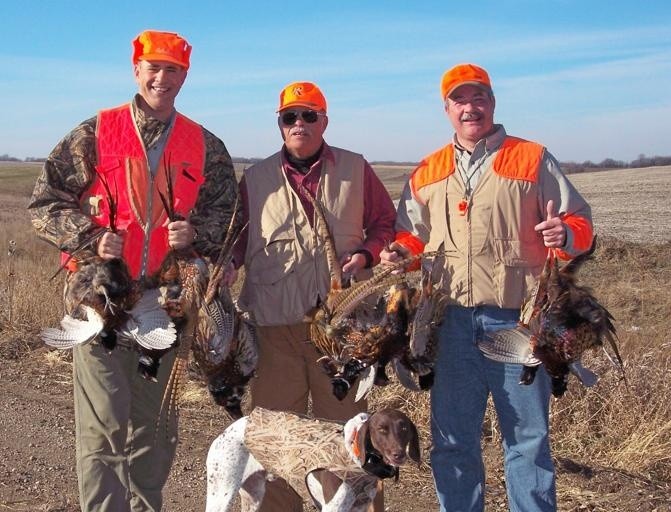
[{"xmin": 282, "ymin": 113, "xmax": 325, "ymax": 125}]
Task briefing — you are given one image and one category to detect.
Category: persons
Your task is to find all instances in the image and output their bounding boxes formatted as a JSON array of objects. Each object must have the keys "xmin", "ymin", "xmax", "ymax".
[
  {"xmin": 217, "ymin": 81, "xmax": 397, "ymax": 511},
  {"xmin": 25, "ymin": 31, "xmax": 233, "ymax": 512},
  {"xmin": 379, "ymin": 63, "xmax": 593, "ymax": 512}
]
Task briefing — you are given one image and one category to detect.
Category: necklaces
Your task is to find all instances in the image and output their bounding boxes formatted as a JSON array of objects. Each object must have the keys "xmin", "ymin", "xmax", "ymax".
[{"xmin": 455, "ymin": 153, "xmax": 494, "ymax": 216}]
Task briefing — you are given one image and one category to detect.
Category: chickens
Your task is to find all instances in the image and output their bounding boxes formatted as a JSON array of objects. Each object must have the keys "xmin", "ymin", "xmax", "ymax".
[
  {"xmin": 154, "ymin": 153, "xmax": 258, "ymax": 420},
  {"xmin": 124, "ymin": 189, "xmax": 204, "ymax": 386},
  {"xmin": 299, "ymin": 181, "xmax": 457, "ymax": 400},
  {"xmin": 475, "ymin": 233, "xmax": 624, "ymax": 408},
  {"xmin": 38, "ymin": 168, "xmax": 132, "ymax": 358}
]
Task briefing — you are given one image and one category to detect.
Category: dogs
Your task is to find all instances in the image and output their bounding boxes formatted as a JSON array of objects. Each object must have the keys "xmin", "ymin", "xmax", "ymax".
[{"xmin": 205, "ymin": 409, "xmax": 421, "ymax": 512}]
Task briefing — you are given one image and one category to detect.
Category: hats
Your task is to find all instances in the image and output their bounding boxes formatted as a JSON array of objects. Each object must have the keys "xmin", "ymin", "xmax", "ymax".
[
  {"xmin": 441, "ymin": 64, "xmax": 491, "ymax": 101},
  {"xmin": 132, "ymin": 31, "xmax": 191, "ymax": 69},
  {"xmin": 275, "ymin": 83, "xmax": 326, "ymax": 113}
]
